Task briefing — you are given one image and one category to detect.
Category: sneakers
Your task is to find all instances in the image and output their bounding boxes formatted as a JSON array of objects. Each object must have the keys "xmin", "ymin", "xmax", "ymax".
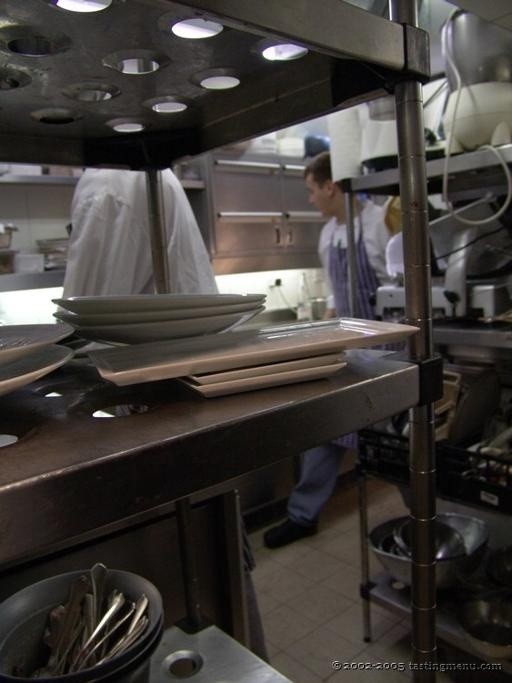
[{"xmin": 262, "ymin": 514, "xmax": 319, "ymax": 550}]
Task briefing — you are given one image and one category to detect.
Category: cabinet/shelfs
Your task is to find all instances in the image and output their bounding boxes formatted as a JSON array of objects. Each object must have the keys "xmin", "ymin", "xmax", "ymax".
[
  {"xmin": 210, "ymin": 154, "xmax": 332, "ymax": 253},
  {"xmin": 347, "ymin": 145, "xmax": 512, "ymax": 673}
]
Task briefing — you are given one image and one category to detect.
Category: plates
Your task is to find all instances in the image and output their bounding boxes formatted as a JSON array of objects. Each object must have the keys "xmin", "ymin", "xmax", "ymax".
[{"xmin": 1, "ymin": 291, "xmax": 421, "ymax": 396}]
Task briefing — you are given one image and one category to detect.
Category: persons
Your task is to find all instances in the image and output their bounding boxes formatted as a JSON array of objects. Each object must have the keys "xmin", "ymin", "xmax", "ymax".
[
  {"xmin": 263, "ymin": 151, "xmax": 407, "ymax": 548},
  {"xmin": 56, "ymin": 169, "xmax": 218, "ymax": 296}
]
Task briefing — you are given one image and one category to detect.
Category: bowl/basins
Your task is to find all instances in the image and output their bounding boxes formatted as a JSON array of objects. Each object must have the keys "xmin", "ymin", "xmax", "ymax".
[{"xmin": 367, "ymin": 511, "xmax": 491, "ymax": 589}]
